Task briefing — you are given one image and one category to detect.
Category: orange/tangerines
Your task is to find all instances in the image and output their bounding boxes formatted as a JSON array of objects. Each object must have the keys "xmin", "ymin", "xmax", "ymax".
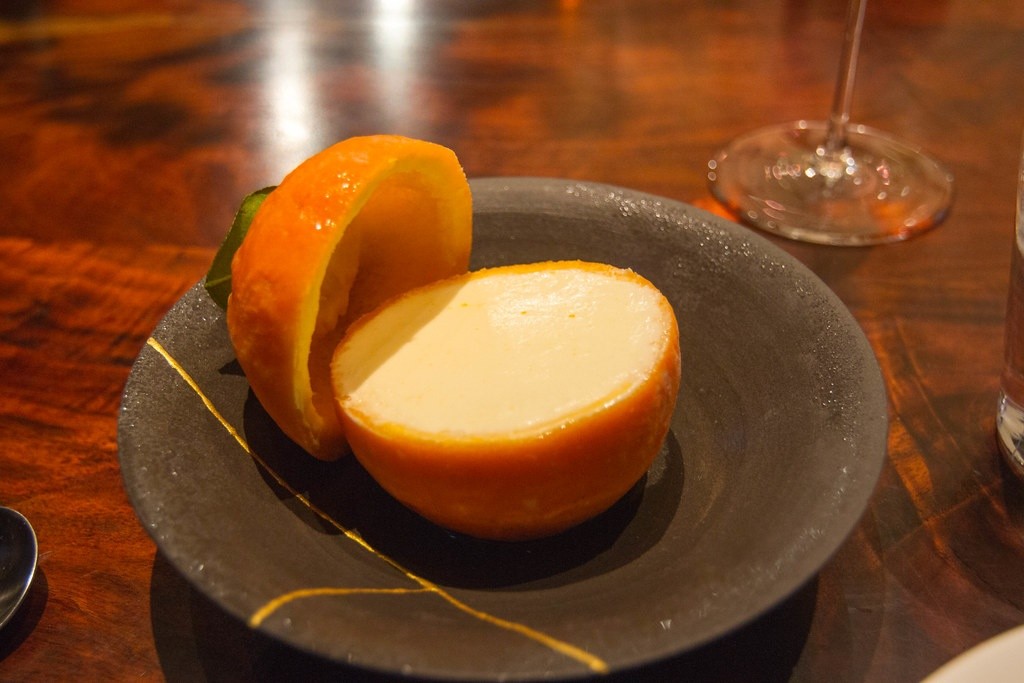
[
  {"xmin": 224, "ymin": 135, "xmax": 471, "ymax": 459},
  {"xmin": 334, "ymin": 258, "xmax": 681, "ymax": 539}
]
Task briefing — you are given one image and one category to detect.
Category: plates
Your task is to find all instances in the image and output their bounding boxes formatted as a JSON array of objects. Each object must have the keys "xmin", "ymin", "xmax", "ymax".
[{"xmin": 119, "ymin": 175, "xmax": 887, "ymax": 679}]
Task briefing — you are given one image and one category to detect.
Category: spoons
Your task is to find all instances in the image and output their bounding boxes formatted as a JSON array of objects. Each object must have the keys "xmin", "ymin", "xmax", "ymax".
[{"xmin": 0, "ymin": 506, "xmax": 38, "ymax": 629}]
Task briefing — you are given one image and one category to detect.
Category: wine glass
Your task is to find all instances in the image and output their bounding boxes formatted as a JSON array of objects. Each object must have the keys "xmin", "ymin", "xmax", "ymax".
[{"xmin": 708, "ymin": 0, "xmax": 957, "ymax": 246}]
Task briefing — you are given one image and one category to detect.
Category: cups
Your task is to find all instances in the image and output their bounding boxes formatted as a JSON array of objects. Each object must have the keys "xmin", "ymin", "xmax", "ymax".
[{"xmin": 998, "ymin": 165, "xmax": 1024, "ymax": 477}]
